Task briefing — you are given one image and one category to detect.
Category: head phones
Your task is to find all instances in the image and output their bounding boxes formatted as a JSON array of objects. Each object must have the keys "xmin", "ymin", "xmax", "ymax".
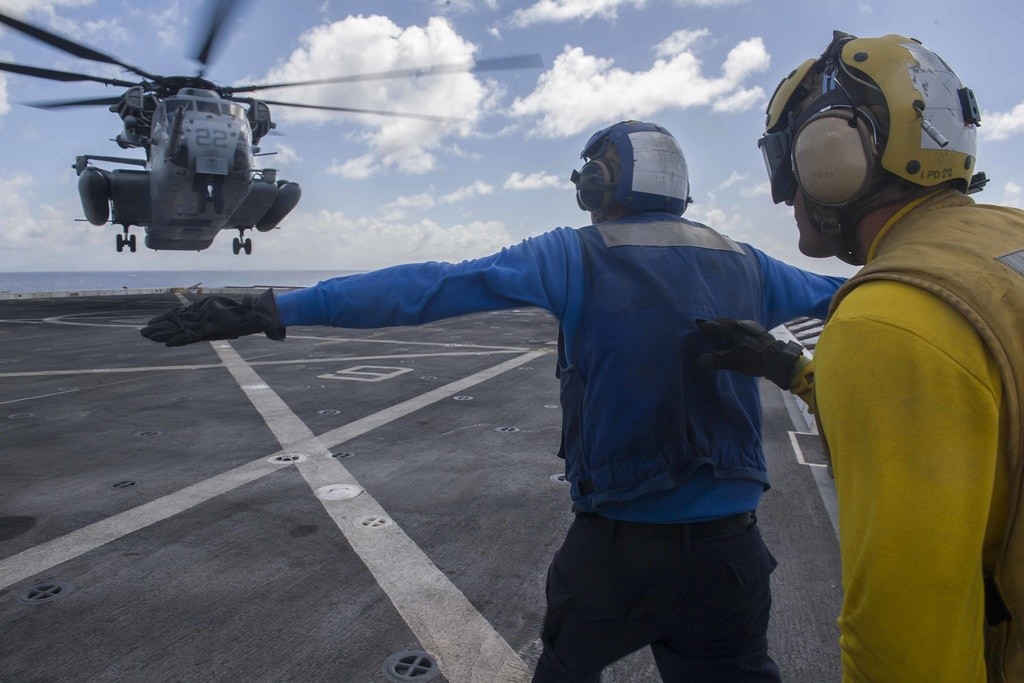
[
  {"xmin": 574, "ymin": 118, "xmax": 642, "ymax": 214},
  {"xmin": 790, "ymin": 37, "xmax": 880, "ymax": 219}
]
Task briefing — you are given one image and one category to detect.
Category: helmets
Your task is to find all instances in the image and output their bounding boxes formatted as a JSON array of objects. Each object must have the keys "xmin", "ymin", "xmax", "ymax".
[
  {"xmin": 766, "ymin": 31, "xmax": 990, "ymax": 207},
  {"xmin": 569, "ymin": 120, "xmax": 694, "ymax": 224}
]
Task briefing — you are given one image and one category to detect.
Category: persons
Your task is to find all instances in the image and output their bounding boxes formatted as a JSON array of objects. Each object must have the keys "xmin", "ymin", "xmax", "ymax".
[
  {"xmin": 141, "ymin": 119, "xmax": 852, "ymax": 683},
  {"xmin": 695, "ymin": 30, "xmax": 1024, "ymax": 683}
]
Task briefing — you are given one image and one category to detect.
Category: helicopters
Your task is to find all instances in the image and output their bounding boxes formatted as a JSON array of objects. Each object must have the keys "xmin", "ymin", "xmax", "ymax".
[{"xmin": 1, "ymin": 0, "xmax": 546, "ymax": 255}]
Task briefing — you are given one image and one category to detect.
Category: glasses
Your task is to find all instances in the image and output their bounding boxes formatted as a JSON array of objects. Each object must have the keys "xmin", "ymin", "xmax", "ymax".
[{"xmin": 757, "ymin": 132, "xmax": 797, "ymax": 204}]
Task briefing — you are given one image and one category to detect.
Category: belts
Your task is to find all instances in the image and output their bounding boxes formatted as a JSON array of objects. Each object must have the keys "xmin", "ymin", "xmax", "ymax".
[{"xmin": 580, "ymin": 512, "xmax": 752, "ymax": 541}]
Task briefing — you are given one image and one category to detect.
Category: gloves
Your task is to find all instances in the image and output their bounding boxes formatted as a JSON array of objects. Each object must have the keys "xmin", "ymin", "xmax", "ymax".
[
  {"xmin": 696, "ymin": 318, "xmax": 804, "ymax": 390},
  {"xmin": 140, "ymin": 286, "xmax": 286, "ymax": 347}
]
